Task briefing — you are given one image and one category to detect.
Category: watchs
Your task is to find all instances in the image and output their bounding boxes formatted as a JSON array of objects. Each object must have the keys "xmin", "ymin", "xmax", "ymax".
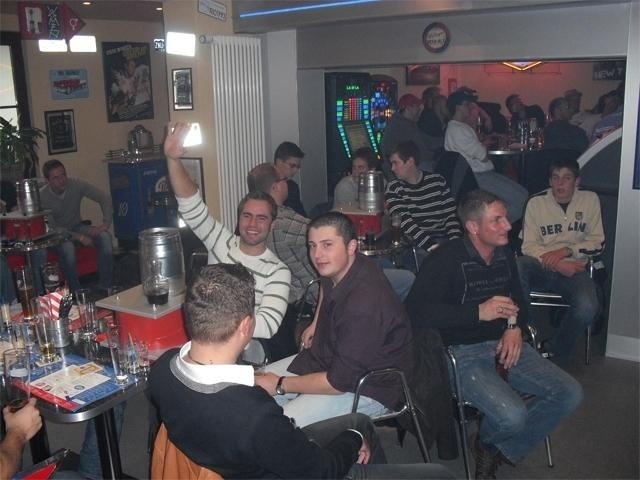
[{"xmin": 275, "ymin": 376, "xmax": 285, "ymax": 397}]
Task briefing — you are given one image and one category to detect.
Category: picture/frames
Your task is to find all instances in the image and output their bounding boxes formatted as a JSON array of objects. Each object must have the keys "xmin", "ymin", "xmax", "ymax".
[
  {"xmin": 178, "ymin": 156, "xmax": 206, "ymax": 204},
  {"xmin": 171, "ymin": 66, "xmax": 195, "ymax": 112},
  {"xmin": 102, "ymin": 41, "xmax": 155, "ymax": 122},
  {"xmin": 44, "ymin": 109, "xmax": 79, "ymax": 156}
]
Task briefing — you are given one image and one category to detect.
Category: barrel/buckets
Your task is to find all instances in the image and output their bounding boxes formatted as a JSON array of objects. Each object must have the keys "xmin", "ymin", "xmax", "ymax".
[
  {"xmin": 138, "ymin": 227, "xmax": 186, "ymax": 297},
  {"xmin": 359, "ymin": 172, "xmax": 384, "ymax": 210},
  {"xmin": 17, "ymin": 180, "xmax": 41, "ymax": 214}
]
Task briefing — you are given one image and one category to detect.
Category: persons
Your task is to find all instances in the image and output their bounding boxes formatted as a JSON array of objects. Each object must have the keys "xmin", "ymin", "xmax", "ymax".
[
  {"xmin": 385, "ymin": 141, "xmax": 464, "ymax": 270},
  {"xmin": 406, "ymin": 190, "xmax": 582, "ymax": 479},
  {"xmin": 383, "ymin": 93, "xmax": 443, "ymax": 174},
  {"xmin": 143, "ymin": 263, "xmax": 461, "ymax": 480},
  {"xmin": 0, "ymin": 399, "xmax": 127, "ymax": 480},
  {"xmin": 332, "ymin": 148, "xmax": 388, "ymax": 237},
  {"xmin": 427, "ymin": 96, "xmax": 449, "ymax": 136},
  {"xmin": 164, "ymin": 122, "xmax": 291, "ymax": 366},
  {"xmin": 515, "ymin": 158, "xmax": 606, "ymax": 358},
  {"xmin": 444, "ymin": 92, "xmax": 529, "ymax": 223},
  {"xmin": 419, "ymin": 88, "xmax": 440, "ymax": 129},
  {"xmin": 40, "ymin": 160, "xmax": 114, "ymax": 298},
  {"xmin": 256, "ymin": 212, "xmax": 415, "ymax": 428},
  {"xmin": 543, "ymin": 98, "xmax": 590, "ymax": 151},
  {"xmin": 247, "ymin": 163, "xmax": 320, "ymax": 314},
  {"xmin": 274, "ymin": 141, "xmax": 307, "ymax": 219},
  {"xmin": 506, "ymin": 94, "xmax": 545, "ymax": 128}
]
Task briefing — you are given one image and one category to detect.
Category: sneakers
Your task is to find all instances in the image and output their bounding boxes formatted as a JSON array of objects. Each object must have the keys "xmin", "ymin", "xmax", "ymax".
[{"xmin": 469, "ymin": 433, "xmax": 496, "ymax": 480}]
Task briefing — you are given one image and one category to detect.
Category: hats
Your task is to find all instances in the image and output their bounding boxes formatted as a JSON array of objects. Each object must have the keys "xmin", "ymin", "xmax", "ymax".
[
  {"xmin": 447, "ymin": 92, "xmax": 479, "ymax": 112},
  {"xmin": 457, "ymin": 87, "xmax": 476, "ymax": 95},
  {"xmin": 399, "ymin": 94, "xmax": 426, "ymax": 109}
]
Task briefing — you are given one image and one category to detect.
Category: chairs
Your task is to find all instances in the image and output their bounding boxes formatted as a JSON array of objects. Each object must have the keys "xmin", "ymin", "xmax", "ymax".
[
  {"xmin": 349, "ymin": 303, "xmax": 433, "ymax": 466},
  {"xmin": 10, "ymin": 448, "xmax": 69, "ymax": 479},
  {"xmin": 436, "ymin": 286, "xmax": 554, "ymax": 478},
  {"xmin": 507, "ymin": 214, "xmax": 608, "ymax": 365},
  {"xmin": 289, "ymin": 276, "xmax": 325, "ymax": 353},
  {"xmin": 159, "ymin": 413, "xmax": 227, "ymax": 480}
]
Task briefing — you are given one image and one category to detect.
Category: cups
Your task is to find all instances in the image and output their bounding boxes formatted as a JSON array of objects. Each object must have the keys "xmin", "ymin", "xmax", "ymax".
[
  {"xmin": 390, "ymin": 213, "xmax": 402, "ymax": 245},
  {"xmin": 74, "ymin": 288, "xmax": 98, "ymax": 334},
  {"xmin": 105, "ymin": 325, "xmax": 130, "ymax": 383},
  {"xmin": 30, "ymin": 295, "xmax": 55, "ymax": 363},
  {"xmin": 2, "ymin": 347, "xmax": 31, "ymax": 409},
  {"xmin": 13, "ymin": 265, "xmax": 39, "ymax": 322},
  {"xmin": 127, "ymin": 341, "xmax": 152, "ymax": 375},
  {"xmin": 507, "ymin": 315, "xmax": 518, "ymax": 329}
]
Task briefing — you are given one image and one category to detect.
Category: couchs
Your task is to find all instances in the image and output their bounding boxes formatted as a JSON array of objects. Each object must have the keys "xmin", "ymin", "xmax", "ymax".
[{"xmin": 10, "ymin": 245, "xmax": 103, "ymax": 287}]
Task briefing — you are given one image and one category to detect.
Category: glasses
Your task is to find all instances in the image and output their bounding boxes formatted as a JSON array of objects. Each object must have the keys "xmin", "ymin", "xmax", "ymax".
[{"xmin": 284, "ymin": 162, "xmax": 300, "ymax": 169}]
